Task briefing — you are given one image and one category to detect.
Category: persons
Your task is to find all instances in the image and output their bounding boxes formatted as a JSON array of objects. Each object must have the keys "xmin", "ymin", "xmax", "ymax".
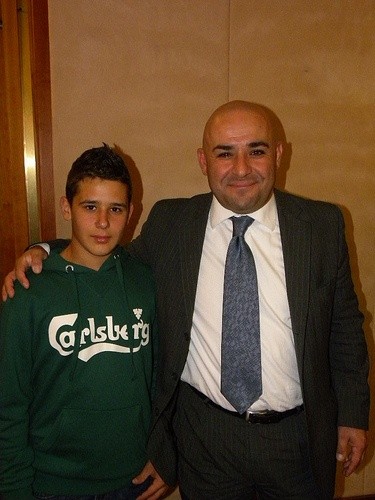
[
  {"xmin": 0, "ymin": 144, "xmax": 176, "ymax": 500},
  {"xmin": 0, "ymin": 98, "xmax": 370, "ymax": 500}
]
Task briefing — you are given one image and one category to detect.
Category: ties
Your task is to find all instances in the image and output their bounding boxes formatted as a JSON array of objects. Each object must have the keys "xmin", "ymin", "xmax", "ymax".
[{"xmin": 220, "ymin": 215, "xmax": 263, "ymax": 413}]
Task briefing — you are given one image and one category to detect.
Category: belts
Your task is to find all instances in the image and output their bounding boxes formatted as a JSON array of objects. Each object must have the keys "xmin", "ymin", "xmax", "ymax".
[{"xmin": 188, "ymin": 383, "xmax": 305, "ymax": 424}]
